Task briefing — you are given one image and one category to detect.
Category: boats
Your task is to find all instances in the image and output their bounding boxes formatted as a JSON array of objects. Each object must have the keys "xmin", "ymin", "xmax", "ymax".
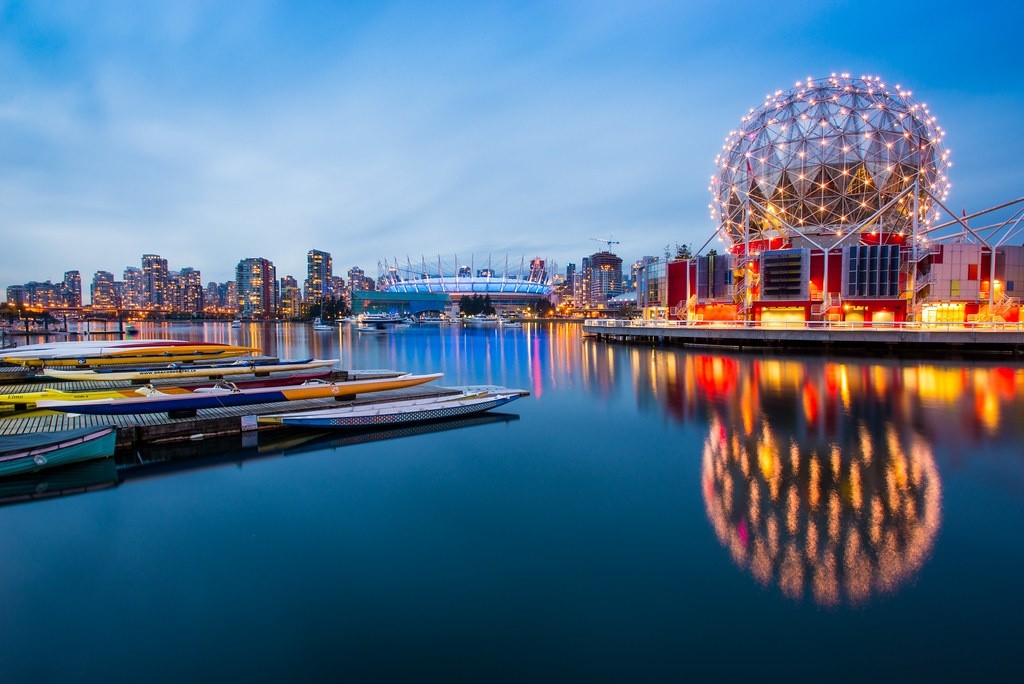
[
  {"xmin": 505, "ymin": 321, "xmax": 523, "ymax": 328},
  {"xmin": 355, "ymin": 326, "xmax": 377, "ymax": 334},
  {"xmin": 125, "ymin": 324, "xmax": 140, "ymax": 336},
  {"xmin": 312, "ymin": 325, "xmax": 336, "ymax": 332},
  {"xmin": 0, "ymin": 332, "xmax": 533, "ymax": 483},
  {"xmin": 230, "ymin": 318, "xmax": 242, "ymax": 328},
  {"xmin": 333, "ymin": 309, "xmax": 498, "ymax": 326}
]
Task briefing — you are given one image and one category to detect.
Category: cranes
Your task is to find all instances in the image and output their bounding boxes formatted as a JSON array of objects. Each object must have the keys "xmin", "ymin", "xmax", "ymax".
[{"xmin": 589, "ymin": 237, "xmax": 621, "ymax": 254}]
